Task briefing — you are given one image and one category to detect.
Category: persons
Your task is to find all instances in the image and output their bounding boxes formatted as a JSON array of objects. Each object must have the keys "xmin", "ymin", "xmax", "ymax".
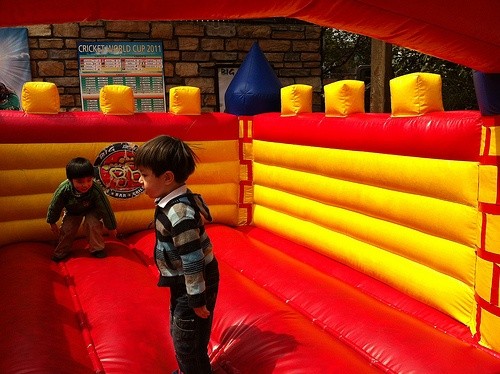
[
  {"xmin": 0, "ymin": 82, "xmax": 20, "ymax": 110},
  {"xmin": 134, "ymin": 135, "xmax": 219, "ymax": 374},
  {"xmin": 46, "ymin": 157, "xmax": 117, "ymax": 263}
]
